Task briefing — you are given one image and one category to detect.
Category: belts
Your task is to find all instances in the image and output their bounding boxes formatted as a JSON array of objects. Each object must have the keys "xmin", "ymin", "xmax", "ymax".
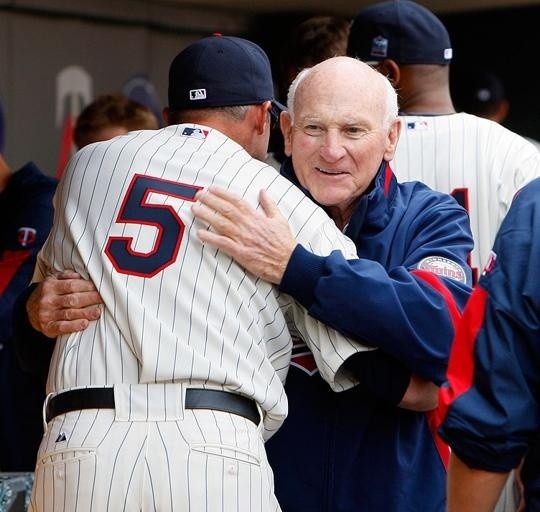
[{"xmin": 44, "ymin": 388, "xmax": 260, "ymax": 426}]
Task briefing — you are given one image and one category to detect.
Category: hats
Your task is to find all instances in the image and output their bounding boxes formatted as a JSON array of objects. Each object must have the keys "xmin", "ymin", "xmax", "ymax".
[
  {"xmin": 169, "ymin": 32, "xmax": 288, "ymax": 117},
  {"xmin": 346, "ymin": 0, "xmax": 453, "ymax": 65}
]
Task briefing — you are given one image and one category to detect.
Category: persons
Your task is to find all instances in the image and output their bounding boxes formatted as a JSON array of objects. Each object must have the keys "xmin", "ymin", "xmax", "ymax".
[
  {"xmin": 1, "ymin": 92, "xmax": 159, "ymax": 473},
  {"xmin": 344, "ymin": 0, "xmax": 540, "ymax": 512}
]
